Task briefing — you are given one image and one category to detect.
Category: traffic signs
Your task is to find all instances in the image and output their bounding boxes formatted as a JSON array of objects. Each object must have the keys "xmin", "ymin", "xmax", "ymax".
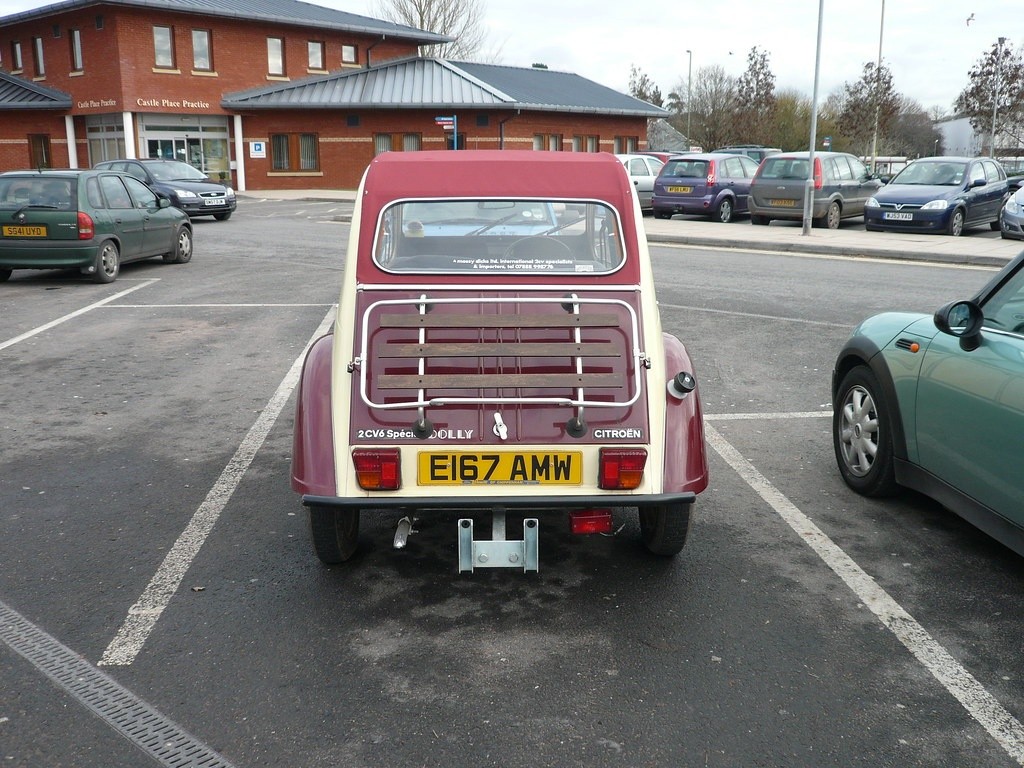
[{"xmin": 435, "ymin": 116, "xmax": 454, "ymax": 129}]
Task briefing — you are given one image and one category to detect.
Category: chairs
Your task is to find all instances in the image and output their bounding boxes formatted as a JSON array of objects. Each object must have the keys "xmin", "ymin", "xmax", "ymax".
[
  {"xmin": 694, "ymin": 165, "xmax": 705, "ymax": 176},
  {"xmin": 793, "ymin": 164, "xmax": 805, "ymax": 176}
]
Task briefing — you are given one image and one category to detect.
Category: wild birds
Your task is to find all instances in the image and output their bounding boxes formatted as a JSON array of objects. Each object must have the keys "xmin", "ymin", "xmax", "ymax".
[{"xmin": 966, "ymin": 13, "xmax": 976, "ymax": 27}]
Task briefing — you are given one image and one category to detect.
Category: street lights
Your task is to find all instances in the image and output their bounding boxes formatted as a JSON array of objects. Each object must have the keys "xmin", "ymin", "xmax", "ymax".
[
  {"xmin": 686, "ymin": 50, "xmax": 692, "ymax": 150},
  {"xmin": 990, "ymin": 37, "xmax": 1005, "ymax": 156}
]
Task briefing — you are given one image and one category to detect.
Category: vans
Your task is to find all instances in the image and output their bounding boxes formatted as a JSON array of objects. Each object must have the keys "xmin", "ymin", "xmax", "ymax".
[{"xmin": 747, "ymin": 152, "xmax": 884, "ymax": 229}]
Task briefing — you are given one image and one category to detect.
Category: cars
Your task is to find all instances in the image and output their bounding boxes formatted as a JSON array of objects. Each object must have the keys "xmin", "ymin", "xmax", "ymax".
[
  {"xmin": 94, "ymin": 158, "xmax": 238, "ymax": 220},
  {"xmin": 832, "ymin": 245, "xmax": 1024, "ymax": 556},
  {"xmin": 863, "ymin": 156, "xmax": 1009, "ymax": 236},
  {"xmin": 290, "ymin": 150, "xmax": 709, "ymax": 564},
  {"xmin": 651, "ymin": 153, "xmax": 760, "ymax": 222},
  {"xmin": 617, "ymin": 154, "xmax": 666, "ymax": 211},
  {"xmin": 0, "ymin": 169, "xmax": 194, "ymax": 284}
]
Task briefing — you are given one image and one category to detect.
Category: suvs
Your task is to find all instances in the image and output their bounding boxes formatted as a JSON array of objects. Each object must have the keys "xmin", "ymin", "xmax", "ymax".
[{"xmin": 711, "ymin": 144, "xmax": 783, "ymax": 164}]
